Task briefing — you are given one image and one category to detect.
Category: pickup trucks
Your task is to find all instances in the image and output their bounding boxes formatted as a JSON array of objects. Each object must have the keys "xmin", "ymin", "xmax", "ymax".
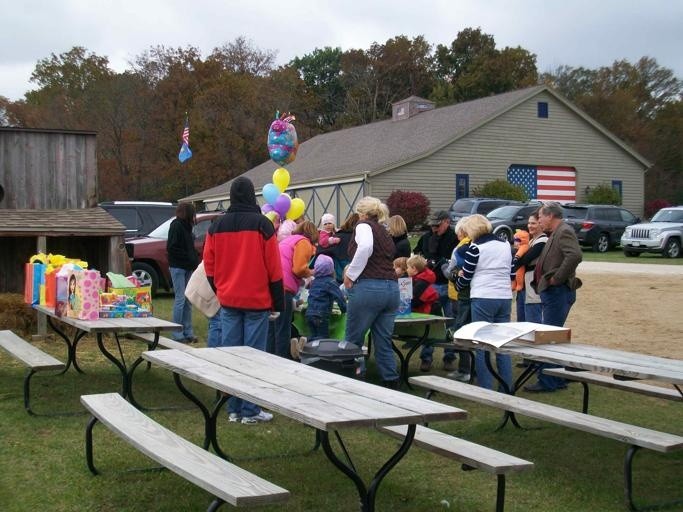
[{"xmin": 125, "ymin": 211, "xmax": 218, "ymax": 293}]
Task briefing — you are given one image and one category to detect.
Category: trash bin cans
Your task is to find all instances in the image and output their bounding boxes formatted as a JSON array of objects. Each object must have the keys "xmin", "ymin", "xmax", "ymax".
[{"xmin": 300, "ymin": 338, "xmax": 363, "ymax": 378}]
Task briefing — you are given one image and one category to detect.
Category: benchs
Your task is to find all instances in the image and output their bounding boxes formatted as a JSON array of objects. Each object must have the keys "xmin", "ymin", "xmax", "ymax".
[{"xmin": 1, "ymin": 328, "xmax": 69, "ymax": 419}]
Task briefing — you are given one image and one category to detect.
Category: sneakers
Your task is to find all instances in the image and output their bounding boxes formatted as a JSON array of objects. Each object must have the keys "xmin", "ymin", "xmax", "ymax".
[
  {"xmin": 420, "ymin": 361, "xmax": 431, "ymax": 371},
  {"xmin": 444, "ymin": 360, "xmax": 455, "ymax": 370},
  {"xmin": 228, "ymin": 413, "xmax": 241, "ymax": 422},
  {"xmin": 524, "ymin": 382, "xmax": 555, "ymax": 392},
  {"xmin": 241, "ymin": 410, "xmax": 273, "ymax": 425},
  {"xmin": 447, "ymin": 370, "xmax": 471, "ymax": 381}
]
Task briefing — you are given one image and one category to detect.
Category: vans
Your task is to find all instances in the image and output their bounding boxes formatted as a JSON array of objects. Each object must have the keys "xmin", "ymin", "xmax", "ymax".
[{"xmin": 448, "ymin": 197, "xmax": 521, "ymax": 227}]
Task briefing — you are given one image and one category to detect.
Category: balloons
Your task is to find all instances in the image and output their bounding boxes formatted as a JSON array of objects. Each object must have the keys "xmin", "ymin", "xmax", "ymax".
[
  {"xmin": 261, "ymin": 165, "xmax": 306, "ymax": 226},
  {"xmin": 268, "ymin": 109, "xmax": 300, "ymax": 167}
]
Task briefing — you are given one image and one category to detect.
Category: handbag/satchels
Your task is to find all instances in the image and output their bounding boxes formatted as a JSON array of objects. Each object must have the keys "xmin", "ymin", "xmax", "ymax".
[{"xmin": 67, "ymin": 269, "xmax": 100, "ymax": 320}]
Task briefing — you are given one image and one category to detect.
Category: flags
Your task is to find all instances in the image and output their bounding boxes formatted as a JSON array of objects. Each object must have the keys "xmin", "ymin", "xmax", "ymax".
[{"xmin": 177, "ymin": 117, "xmax": 193, "ymax": 164}]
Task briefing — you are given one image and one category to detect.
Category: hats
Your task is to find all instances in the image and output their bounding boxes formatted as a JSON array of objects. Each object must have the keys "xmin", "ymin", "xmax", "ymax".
[
  {"xmin": 426, "ymin": 210, "xmax": 449, "ymax": 226},
  {"xmin": 321, "ymin": 214, "xmax": 334, "ymax": 225},
  {"xmin": 314, "ymin": 254, "xmax": 334, "ymax": 276}
]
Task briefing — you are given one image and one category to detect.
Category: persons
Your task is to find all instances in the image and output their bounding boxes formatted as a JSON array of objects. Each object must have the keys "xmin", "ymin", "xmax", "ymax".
[
  {"xmin": 204, "ymin": 176, "xmax": 284, "ymax": 427},
  {"xmin": 290, "ymin": 197, "xmax": 411, "ymax": 391},
  {"xmin": 166, "ymin": 201, "xmax": 199, "ymax": 343},
  {"xmin": 415, "ymin": 201, "xmax": 583, "ymax": 395},
  {"xmin": 267, "ymin": 221, "xmax": 319, "ymax": 360}
]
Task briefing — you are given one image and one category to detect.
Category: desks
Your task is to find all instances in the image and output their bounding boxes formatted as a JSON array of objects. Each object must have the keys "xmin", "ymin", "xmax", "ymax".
[{"xmin": 453, "ymin": 326, "xmax": 682, "ymax": 512}]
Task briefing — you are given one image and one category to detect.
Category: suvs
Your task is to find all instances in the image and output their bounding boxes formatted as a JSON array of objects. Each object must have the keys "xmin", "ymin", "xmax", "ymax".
[
  {"xmin": 99, "ymin": 200, "xmax": 177, "ymax": 235},
  {"xmin": 622, "ymin": 203, "xmax": 683, "ymax": 259}
]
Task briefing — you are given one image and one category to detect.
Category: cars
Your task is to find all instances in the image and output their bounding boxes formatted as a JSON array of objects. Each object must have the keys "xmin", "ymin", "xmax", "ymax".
[
  {"xmin": 485, "ymin": 205, "xmax": 537, "ymax": 240},
  {"xmin": 562, "ymin": 203, "xmax": 639, "ymax": 251}
]
